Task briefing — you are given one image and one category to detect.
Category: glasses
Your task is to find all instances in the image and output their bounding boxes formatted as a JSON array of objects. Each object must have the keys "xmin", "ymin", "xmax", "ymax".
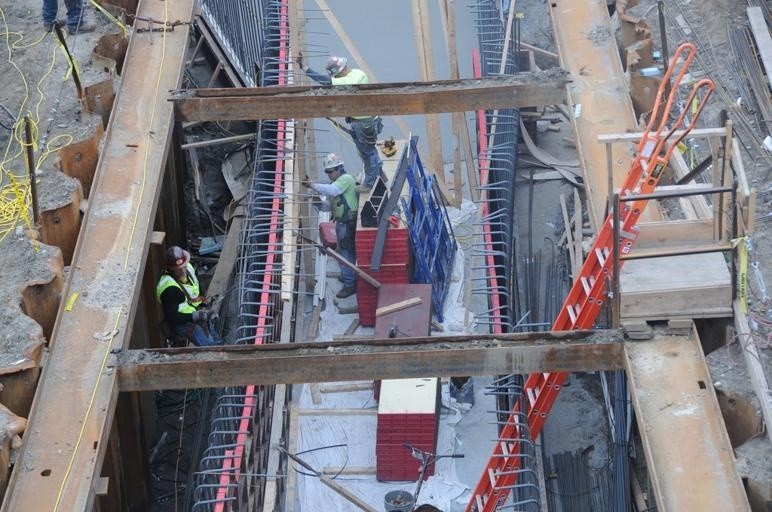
[{"xmin": 324, "ymin": 169, "xmax": 336, "ymax": 174}]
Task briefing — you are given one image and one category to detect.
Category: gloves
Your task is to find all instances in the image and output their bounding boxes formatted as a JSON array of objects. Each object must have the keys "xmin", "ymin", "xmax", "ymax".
[
  {"xmin": 301, "ymin": 176, "xmax": 313, "ymax": 188},
  {"xmin": 191, "ymin": 309, "xmax": 208, "ymax": 322},
  {"xmin": 295, "ymin": 53, "xmax": 306, "ymax": 70}
]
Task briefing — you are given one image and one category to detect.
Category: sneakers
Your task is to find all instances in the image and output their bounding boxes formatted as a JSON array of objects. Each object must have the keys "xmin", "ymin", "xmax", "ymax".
[
  {"xmin": 335, "ymin": 286, "xmax": 356, "ymax": 298},
  {"xmin": 354, "ymin": 182, "xmax": 373, "ymax": 193}
]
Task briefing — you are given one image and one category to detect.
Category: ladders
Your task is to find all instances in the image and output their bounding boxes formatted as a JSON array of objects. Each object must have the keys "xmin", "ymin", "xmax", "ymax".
[{"xmin": 465, "ymin": 43, "xmax": 715, "ymax": 511}]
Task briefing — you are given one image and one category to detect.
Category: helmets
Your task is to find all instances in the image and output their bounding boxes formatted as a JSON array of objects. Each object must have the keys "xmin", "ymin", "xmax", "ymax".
[
  {"xmin": 321, "ymin": 153, "xmax": 344, "ymax": 169},
  {"xmin": 324, "ymin": 56, "xmax": 348, "ymax": 78},
  {"xmin": 164, "ymin": 246, "xmax": 191, "ymax": 271}
]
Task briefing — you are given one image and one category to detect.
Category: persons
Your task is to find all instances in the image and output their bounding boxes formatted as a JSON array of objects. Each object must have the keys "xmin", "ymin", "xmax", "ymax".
[
  {"xmin": 156, "ymin": 245, "xmax": 224, "ymax": 348},
  {"xmin": 296, "ymin": 53, "xmax": 388, "ymax": 193},
  {"xmin": 302, "ymin": 153, "xmax": 358, "ymax": 298},
  {"xmin": 42, "ymin": 0, "xmax": 96, "ymax": 35}
]
